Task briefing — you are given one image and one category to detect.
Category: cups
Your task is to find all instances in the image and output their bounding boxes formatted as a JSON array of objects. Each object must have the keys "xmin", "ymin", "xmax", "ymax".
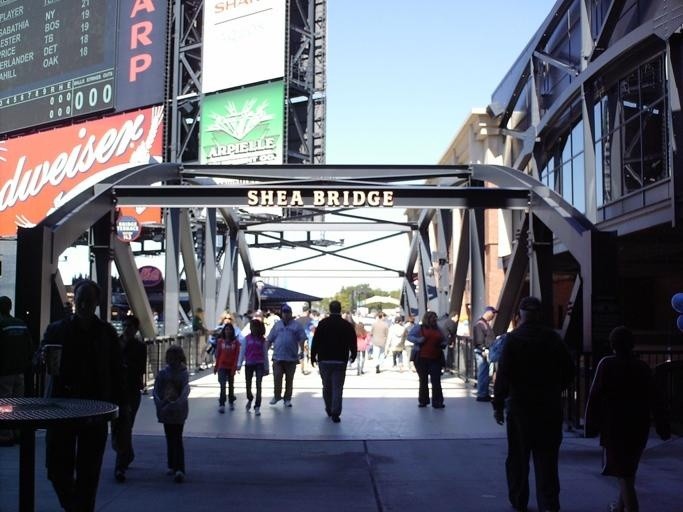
[{"xmin": 44, "ymin": 344, "xmax": 61, "ymax": 376}]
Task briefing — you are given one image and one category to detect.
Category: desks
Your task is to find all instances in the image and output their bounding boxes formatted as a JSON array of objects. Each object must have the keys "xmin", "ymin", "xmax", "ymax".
[{"xmin": 0, "ymin": 396, "xmax": 119, "ymax": 511}]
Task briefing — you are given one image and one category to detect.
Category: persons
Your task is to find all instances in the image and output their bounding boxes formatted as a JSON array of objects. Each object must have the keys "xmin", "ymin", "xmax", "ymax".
[
  {"xmin": 235, "ymin": 317, "xmax": 271, "ymax": 417},
  {"xmin": 309, "ymin": 299, "xmax": 358, "ymax": 424},
  {"xmin": 151, "ymin": 344, "xmax": 191, "ymax": 483},
  {"xmin": 108, "ymin": 315, "xmax": 148, "ymax": 483},
  {"xmin": 211, "ymin": 322, "xmax": 242, "ymax": 415},
  {"xmin": 191, "ymin": 307, "xmax": 211, "ymax": 369},
  {"xmin": 30, "ymin": 277, "xmax": 128, "ymax": 511},
  {"xmin": 0, "ymin": 294, "xmax": 38, "ymax": 448},
  {"xmin": 216, "ymin": 305, "xmax": 422, "ymax": 376},
  {"xmin": 264, "ymin": 303, "xmax": 309, "ymax": 408},
  {"xmin": 406, "ymin": 310, "xmax": 450, "ymax": 410},
  {"xmin": 582, "ymin": 323, "xmax": 673, "ymax": 511},
  {"xmin": 470, "ymin": 306, "xmax": 500, "ymax": 403},
  {"xmin": 491, "ymin": 296, "xmax": 580, "ymax": 511}
]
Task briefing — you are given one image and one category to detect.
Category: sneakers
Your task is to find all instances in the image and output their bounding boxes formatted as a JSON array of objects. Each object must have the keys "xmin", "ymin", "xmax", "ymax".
[
  {"xmin": 476, "ymin": 395, "xmax": 495, "ymax": 402},
  {"xmin": 328, "ymin": 414, "xmax": 341, "ymax": 423},
  {"xmin": 217, "ymin": 403, "xmax": 225, "ymax": 414},
  {"xmin": 229, "ymin": 403, "xmax": 234, "ymax": 411},
  {"xmin": 164, "ymin": 467, "xmax": 185, "ymax": 483},
  {"xmin": 607, "ymin": 501, "xmax": 618, "ymax": 512},
  {"xmin": 113, "ymin": 457, "xmax": 135, "ymax": 483},
  {"xmin": 269, "ymin": 396, "xmax": 282, "ymax": 405},
  {"xmin": 254, "ymin": 405, "xmax": 261, "ymax": 415},
  {"xmin": 284, "ymin": 399, "xmax": 292, "ymax": 407},
  {"xmin": 418, "ymin": 401, "xmax": 445, "ymax": 408},
  {"xmin": 245, "ymin": 399, "xmax": 253, "ymax": 410}
]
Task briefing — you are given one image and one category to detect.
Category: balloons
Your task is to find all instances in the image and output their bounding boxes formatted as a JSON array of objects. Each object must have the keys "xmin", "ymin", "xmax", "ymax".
[
  {"xmin": 671, "ymin": 292, "xmax": 683, "ymax": 315},
  {"xmin": 676, "ymin": 315, "xmax": 682, "ymax": 331}
]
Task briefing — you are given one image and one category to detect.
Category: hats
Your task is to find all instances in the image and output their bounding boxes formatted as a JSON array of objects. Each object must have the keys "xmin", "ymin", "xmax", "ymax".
[
  {"xmin": 484, "ymin": 306, "xmax": 500, "ymax": 314},
  {"xmin": 282, "ymin": 305, "xmax": 291, "ymax": 313},
  {"xmin": 519, "ymin": 296, "xmax": 543, "ymax": 314}
]
